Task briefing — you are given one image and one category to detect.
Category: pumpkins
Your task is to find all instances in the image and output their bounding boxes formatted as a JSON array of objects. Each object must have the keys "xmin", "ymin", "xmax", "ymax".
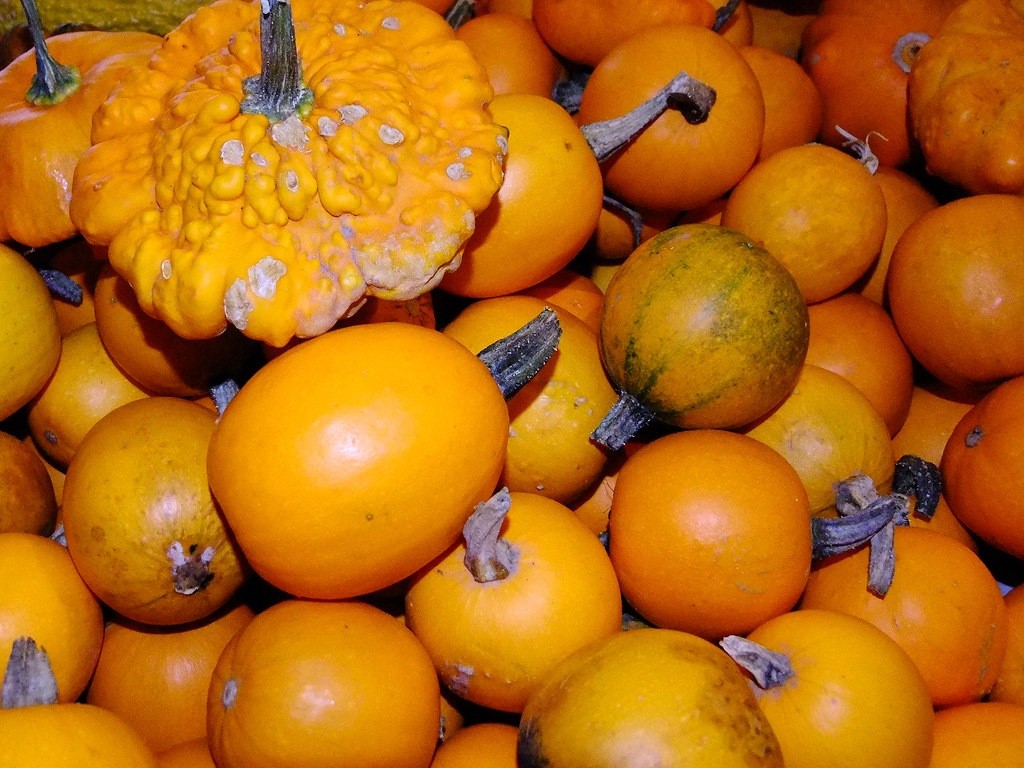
[{"xmin": 0, "ymin": 0, "xmax": 1024, "ymax": 768}]
